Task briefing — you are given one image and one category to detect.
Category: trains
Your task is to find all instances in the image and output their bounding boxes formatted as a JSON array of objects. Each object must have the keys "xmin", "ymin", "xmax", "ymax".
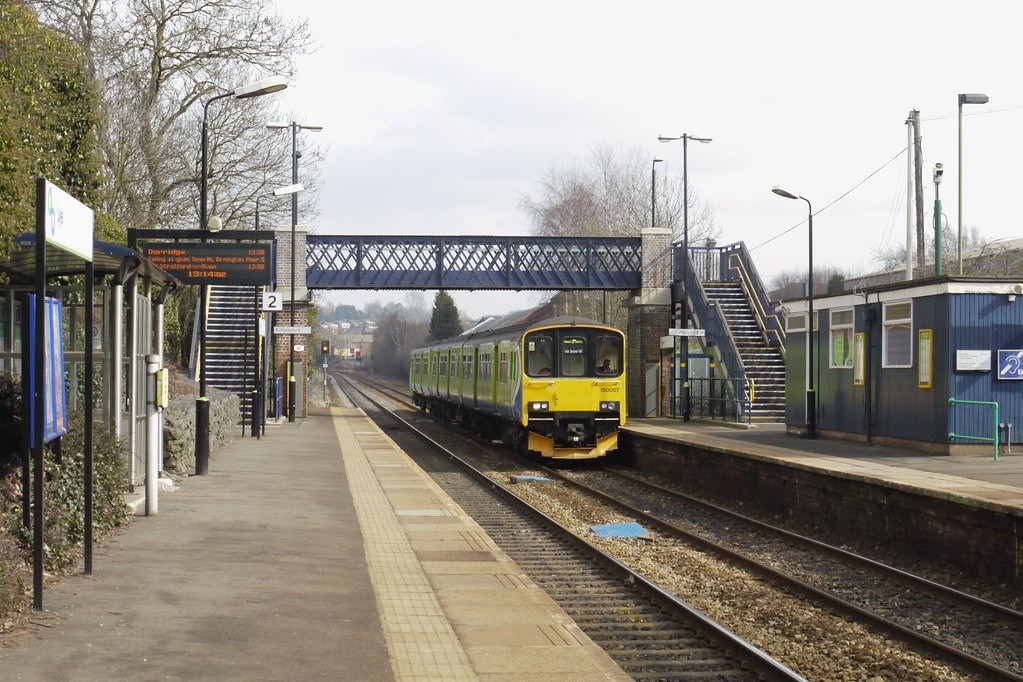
[{"xmin": 407, "ymin": 314, "xmax": 629, "ymax": 466}]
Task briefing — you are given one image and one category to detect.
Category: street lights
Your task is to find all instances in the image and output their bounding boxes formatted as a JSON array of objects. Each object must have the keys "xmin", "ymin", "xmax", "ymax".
[
  {"xmin": 193, "ymin": 75, "xmax": 288, "ymax": 476},
  {"xmin": 651, "ymin": 158, "xmax": 664, "ymax": 228},
  {"xmin": 265, "ymin": 121, "xmax": 324, "ymax": 424},
  {"xmin": 657, "ymin": 133, "xmax": 712, "ymax": 422},
  {"xmin": 769, "ymin": 184, "xmax": 817, "ymax": 438},
  {"xmin": 252, "ymin": 181, "xmax": 305, "ymax": 440},
  {"xmin": 956, "ymin": 93, "xmax": 990, "ymax": 276}
]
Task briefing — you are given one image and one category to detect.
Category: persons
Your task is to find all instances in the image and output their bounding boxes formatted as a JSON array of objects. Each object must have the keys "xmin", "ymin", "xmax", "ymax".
[{"xmin": 596, "ymin": 359, "xmax": 614, "ymax": 374}]
